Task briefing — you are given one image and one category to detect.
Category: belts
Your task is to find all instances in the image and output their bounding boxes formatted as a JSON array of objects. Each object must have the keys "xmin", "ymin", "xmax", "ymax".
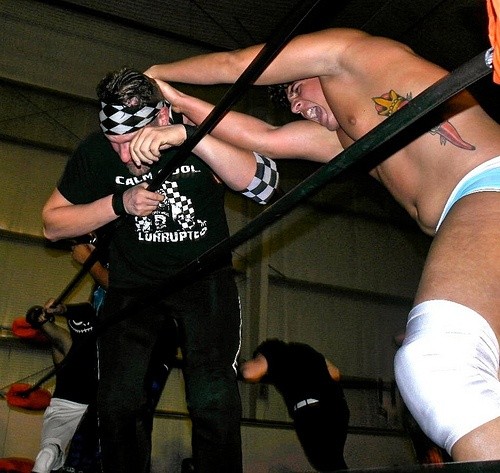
[{"xmin": 294, "ymin": 398, "xmax": 319, "ymax": 411}]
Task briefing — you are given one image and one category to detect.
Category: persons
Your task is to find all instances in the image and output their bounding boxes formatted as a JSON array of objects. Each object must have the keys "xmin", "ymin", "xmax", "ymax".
[
  {"xmin": 40, "ymin": 67, "xmax": 278, "ymax": 473},
  {"xmin": 239, "ymin": 336, "xmax": 351, "ymax": 472},
  {"xmin": 25, "ymin": 240, "xmax": 112, "ymax": 473},
  {"xmin": 142, "ymin": 28, "xmax": 500, "ymax": 462}
]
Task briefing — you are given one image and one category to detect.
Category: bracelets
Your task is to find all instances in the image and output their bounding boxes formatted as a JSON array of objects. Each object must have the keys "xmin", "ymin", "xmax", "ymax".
[{"xmin": 112, "ymin": 191, "xmax": 128, "ymax": 216}]
